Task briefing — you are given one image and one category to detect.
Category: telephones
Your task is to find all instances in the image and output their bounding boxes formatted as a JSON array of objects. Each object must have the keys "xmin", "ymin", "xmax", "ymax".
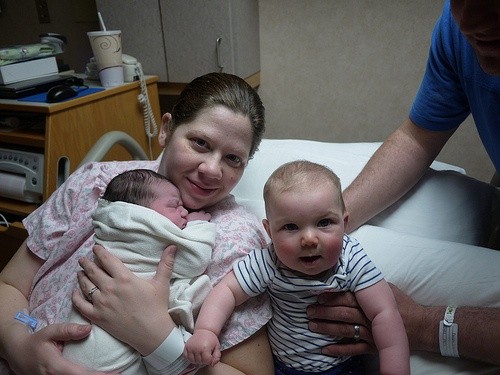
[{"xmin": 87, "ymin": 54, "xmax": 141, "ymax": 81}]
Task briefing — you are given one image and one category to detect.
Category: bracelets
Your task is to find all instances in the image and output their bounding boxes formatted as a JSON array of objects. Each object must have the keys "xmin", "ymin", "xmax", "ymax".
[
  {"xmin": 142, "ymin": 324, "xmax": 210, "ymax": 375},
  {"xmin": 439, "ymin": 306, "xmax": 460, "ymax": 358}
]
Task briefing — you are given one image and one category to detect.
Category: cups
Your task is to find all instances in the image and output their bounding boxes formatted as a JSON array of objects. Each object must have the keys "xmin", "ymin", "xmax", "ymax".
[{"xmin": 86, "ymin": 30, "xmax": 123, "ymax": 87}]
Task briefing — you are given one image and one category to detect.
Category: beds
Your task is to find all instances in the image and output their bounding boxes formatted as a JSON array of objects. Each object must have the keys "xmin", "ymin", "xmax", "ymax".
[{"xmin": 79, "ymin": 132, "xmax": 500, "ymax": 375}]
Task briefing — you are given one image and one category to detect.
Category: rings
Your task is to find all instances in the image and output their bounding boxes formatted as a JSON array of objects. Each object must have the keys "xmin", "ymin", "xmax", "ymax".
[
  {"xmin": 354, "ymin": 324, "xmax": 360, "ymax": 341},
  {"xmin": 87, "ymin": 286, "xmax": 99, "ymax": 303}
]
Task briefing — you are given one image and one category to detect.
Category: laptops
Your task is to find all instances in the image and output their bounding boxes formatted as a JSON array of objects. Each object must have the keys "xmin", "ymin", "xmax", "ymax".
[{"xmin": 0, "ymin": 74, "xmax": 83, "ymax": 99}]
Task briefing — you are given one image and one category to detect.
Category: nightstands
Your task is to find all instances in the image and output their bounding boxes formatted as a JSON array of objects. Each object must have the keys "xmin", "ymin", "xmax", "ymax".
[{"xmin": 0, "ymin": 73, "xmax": 162, "ymax": 270}]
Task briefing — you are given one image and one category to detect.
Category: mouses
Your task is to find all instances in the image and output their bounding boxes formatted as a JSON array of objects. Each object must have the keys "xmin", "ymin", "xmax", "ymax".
[{"xmin": 46, "ymin": 86, "xmax": 78, "ymax": 103}]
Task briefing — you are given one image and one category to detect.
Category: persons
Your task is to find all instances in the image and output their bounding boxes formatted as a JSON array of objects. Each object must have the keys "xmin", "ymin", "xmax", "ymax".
[
  {"xmin": 305, "ymin": 0, "xmax": 500, "ymax": 369},
  {"xmin": 61, "ymin": 169, "xmax": 217, "ymax": 375},
  {"xmin": 1, "ymin": 71, "xmax": 273, "ymax": 375},
  {"xmin": 183, "ymin": 160, "xmax": 411, "ymax": 375}
]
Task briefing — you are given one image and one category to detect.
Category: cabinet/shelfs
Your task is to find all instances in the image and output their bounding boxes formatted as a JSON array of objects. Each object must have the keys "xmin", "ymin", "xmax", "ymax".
[{"xmin": 95, "ymin": 0, "xmax": 260, "ymax": 95}]
